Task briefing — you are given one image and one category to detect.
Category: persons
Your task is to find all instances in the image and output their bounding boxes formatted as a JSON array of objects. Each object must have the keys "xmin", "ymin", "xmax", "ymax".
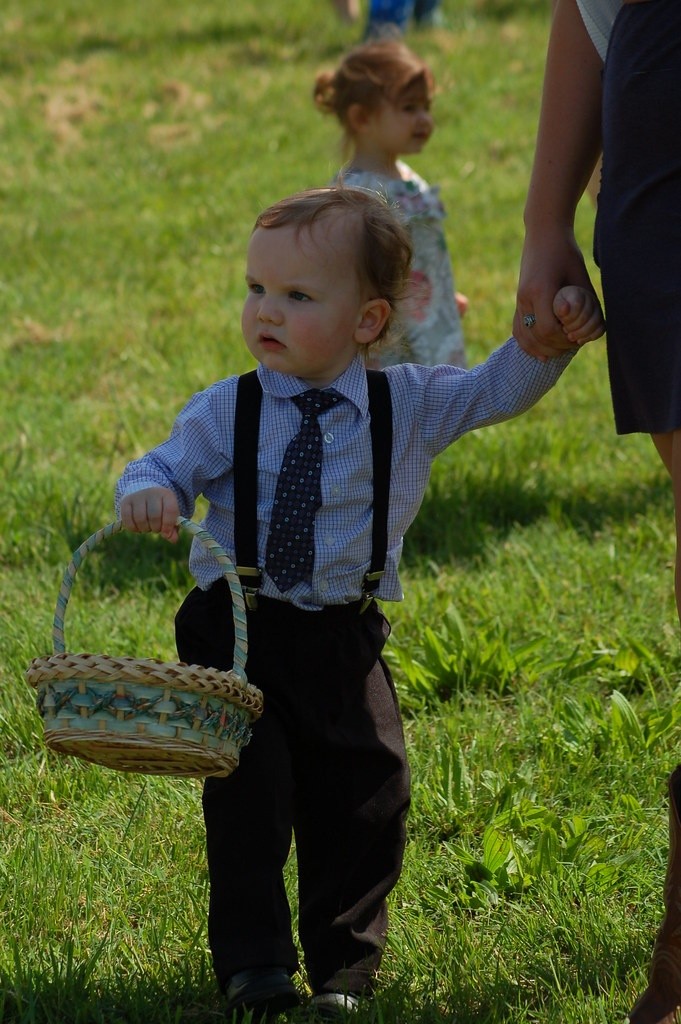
[
  {"xmin": 101, "ymin": 181, "xmax": 612, "ymax": 1022},
  {"xmin": 310, "ymin": 39, "xmax": 472, "ymax": 373},
  {"xmin": 504, "ymin": 3, "xmax": 681, "ymax": 1022},
  {"xmin": 340, "ymin": 0, "xmax": 446, "ymax": 40}
]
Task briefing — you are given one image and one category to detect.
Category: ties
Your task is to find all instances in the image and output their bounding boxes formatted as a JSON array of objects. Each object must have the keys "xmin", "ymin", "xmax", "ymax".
[{"xmin": 267, "ymin": 388, "xmax": 345, "ymax": 595}]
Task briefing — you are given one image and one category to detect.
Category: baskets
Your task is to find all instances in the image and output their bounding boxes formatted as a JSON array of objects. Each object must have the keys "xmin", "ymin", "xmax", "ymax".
[{"xmin": 29, "ymin": 514, "xmax": 263, "ymax": 777}]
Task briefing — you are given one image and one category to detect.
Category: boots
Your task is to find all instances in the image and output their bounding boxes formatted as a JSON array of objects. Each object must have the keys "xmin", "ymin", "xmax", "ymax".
[{"xmin": 611, "ymin": 765, "xmax": 681, "ymax": 1024}]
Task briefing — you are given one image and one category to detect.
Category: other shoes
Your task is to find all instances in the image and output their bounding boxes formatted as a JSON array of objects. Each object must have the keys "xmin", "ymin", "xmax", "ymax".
[
  {"xmin": 226, "ymin": 973, "xmax": 303, "ymax": 1021},
  {"xmin": 312, "ymin": 990, "xmax": 365, "ymax": 1019}
]
